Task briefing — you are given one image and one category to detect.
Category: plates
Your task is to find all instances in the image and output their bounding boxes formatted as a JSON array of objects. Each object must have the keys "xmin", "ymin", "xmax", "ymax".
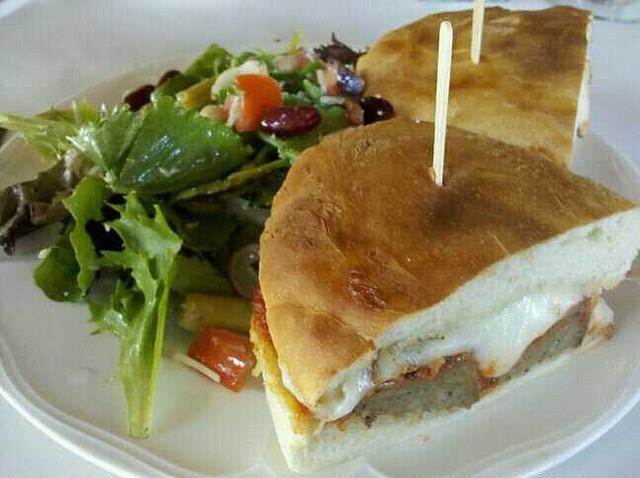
[{"xmin": 0, "ymin": 40, "xmax": 639, "ymax": 477}]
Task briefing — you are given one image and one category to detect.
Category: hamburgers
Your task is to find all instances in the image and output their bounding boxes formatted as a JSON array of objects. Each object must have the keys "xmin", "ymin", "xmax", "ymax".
[
  {"xmin": 353, "ymin": 5, "xmax": 591, "ymax": 172},
  {"xmin": 250, "ymin": 116, "xmax": 640, "ymax": 474}
]
